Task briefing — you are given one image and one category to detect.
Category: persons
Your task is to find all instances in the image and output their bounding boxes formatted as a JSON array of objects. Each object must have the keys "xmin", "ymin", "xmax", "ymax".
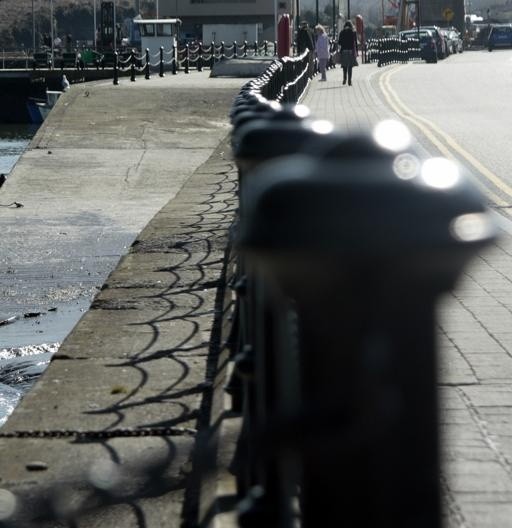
[
  {"xmin": 41, "ymin": 32, "xmax": 51, "ymax": 50},
  {"xmin": 314, "ymin": 22, "xmax": 330, "ymax": 82},
  {"xmin": 54, "ymin": 32, "xmax": 63, "ymax": 50},
  {"xmin": 338, "ymin": 20, "xmax": 359, "ymax": 86},
  {"xmin": 296, "ymin": 21, "xmax": 314, "ymax": 80}
]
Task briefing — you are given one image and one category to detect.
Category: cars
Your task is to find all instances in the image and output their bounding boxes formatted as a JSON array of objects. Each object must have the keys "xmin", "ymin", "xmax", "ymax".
[
  {"xmin": 487, "ymin": 24, "xmax": 512, "ymax": 51},
  {"xmin": 398, "ymin": 26, "xmax": 463, "ymax": 63}
]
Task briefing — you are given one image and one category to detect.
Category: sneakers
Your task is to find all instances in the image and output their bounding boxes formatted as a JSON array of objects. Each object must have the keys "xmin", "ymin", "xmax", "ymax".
[
  {"xmin": 343, "ymin": 80, "xmax": 352, "ymax": 86},
  {"xmin": 318, "ymin": 77, "xmax": 326, "ymax": 81}
]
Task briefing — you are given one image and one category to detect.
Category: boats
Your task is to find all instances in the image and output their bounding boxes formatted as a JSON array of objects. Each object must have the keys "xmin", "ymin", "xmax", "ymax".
[{"xmin": 27, "ymin": 90, "xmax": 62, "ymax": 124}]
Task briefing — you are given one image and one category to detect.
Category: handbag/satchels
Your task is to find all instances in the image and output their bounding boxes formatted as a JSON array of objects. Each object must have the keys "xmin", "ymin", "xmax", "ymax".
[{"xmin": 353, "ymin": 45, "xmax": 358, "ymax": 58}]
